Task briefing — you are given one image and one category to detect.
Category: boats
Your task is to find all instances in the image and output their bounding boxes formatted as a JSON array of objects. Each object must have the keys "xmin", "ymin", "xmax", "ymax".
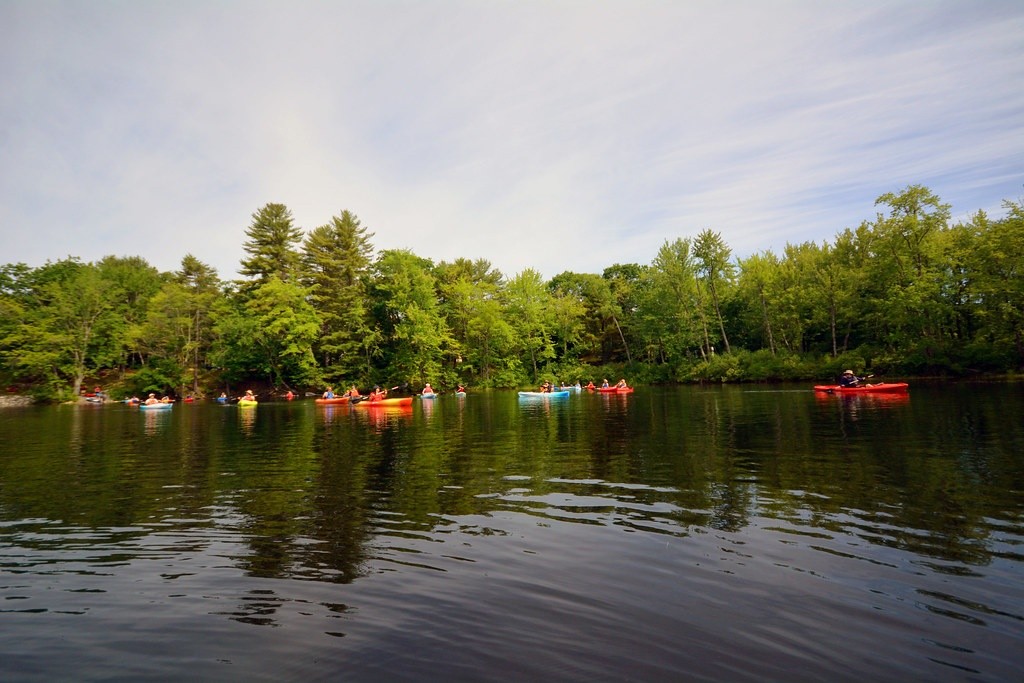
[
  {"xmin": 584, "ymin": 385, "xmax": 596, "ymax": 390},
  {"xmin": 450, "ymin": 392, "xmax": 466, "ymax": 398},
  {"xmin": 561, "ymin": 387, "xmax": 582, "ymax": 391},
  {"xmin": 596, "ymin": 387, "xmax": 634, "ymax": 393},
  {"xmin": 518, "ymin": 392, "xmax": 570, "ymax": 398},
  {"xmin": 315, "ymin": 396, "xmax": 351, "ymax": 405},
  {"xmin": 237, "ymin": 399, "xmax": 258, "ymax": 406},
  {"xmin": 139, "ymin": 402, "xmax": 173, "ymax": 409},
  {"xmin": 217, "ymin": 397, "xmax": 228, "ymax": 401},
  {"xmin": 351, "ymin": 396, "xmax": 364, "ymax": 404},
  {"xmin": 359, "ymin": 399, "xmax": 413, "ymax": 406},
  {"xmin": 420, "ymin": 393, "xmax": 437, "ymax": 399},
  {"xmin": 814, "ymin": 382, "xmax": 908, "ymax": 395}
]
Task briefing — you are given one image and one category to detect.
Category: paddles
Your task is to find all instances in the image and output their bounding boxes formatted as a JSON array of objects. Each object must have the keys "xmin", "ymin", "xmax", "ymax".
[
  {"xmin": 352, "ymin": 382, "xmax": 407, "ymax": 403},
  {"xmin": 305, "ymin": 391, "xmax": 344, "ymax": 397},
  {"xmin": 823, "ymin": 374, "xmax": 875, "ymax": 395}
]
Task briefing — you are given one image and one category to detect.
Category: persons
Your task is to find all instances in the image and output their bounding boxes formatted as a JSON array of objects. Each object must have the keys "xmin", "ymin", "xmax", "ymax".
[
  {"xmin": 95, "ymin": 385, "xmax": 100, "ymax": 402},
  {"xmin": 221, "ymin": 392, "xmax": 227, "ymax": 398},
  {"xmin": 421, "ymin": 383, "xmax": 433, "ymax": 395},
  {"xmin": 241, "ymin": 390, "xmax": 255, "ymax": 401},
  {"xmin": 145, "ymin": 393, "xmax": 158, "ymax": 405},
  {"xmin": 841, "ymin": 369, "xmax": 874, "ymax": 388},
  {"xmin": 322, "ymin": 386, "xmax": 338, "ymax": 400},
  {"xmin": 369, "ymin": 384, "xmax": 387, "ymax": 402},
  {"xmin": 539, "ymin": 377, "xmax": 627, "ymax": 393},
  {"xmin": 342, "ymin": 385, "xmax": 359, "ymax": 398},
  {"xmin": 457, "ymin": 383, "xmax": 466, "ymax": 392}
]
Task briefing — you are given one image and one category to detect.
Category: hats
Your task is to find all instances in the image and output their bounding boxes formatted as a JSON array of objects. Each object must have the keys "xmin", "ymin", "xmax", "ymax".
[
  {"xmin": 245, "ymin": 389, "xmax": 253, "ymax": 395},
  {"xmin": 149, "ymin": 393, "xmax": 155, "ymax": 397},
  {"xmin": 373, "ymin": 385, "xmax": 380, "ymax": 390},
  {"xmin": 426, "ymin": 383, "xmax": 430, "ymax": 386},
  {"xmin": 844, "ymin": 370, "xmax": 853, "ymax": 375}
]
